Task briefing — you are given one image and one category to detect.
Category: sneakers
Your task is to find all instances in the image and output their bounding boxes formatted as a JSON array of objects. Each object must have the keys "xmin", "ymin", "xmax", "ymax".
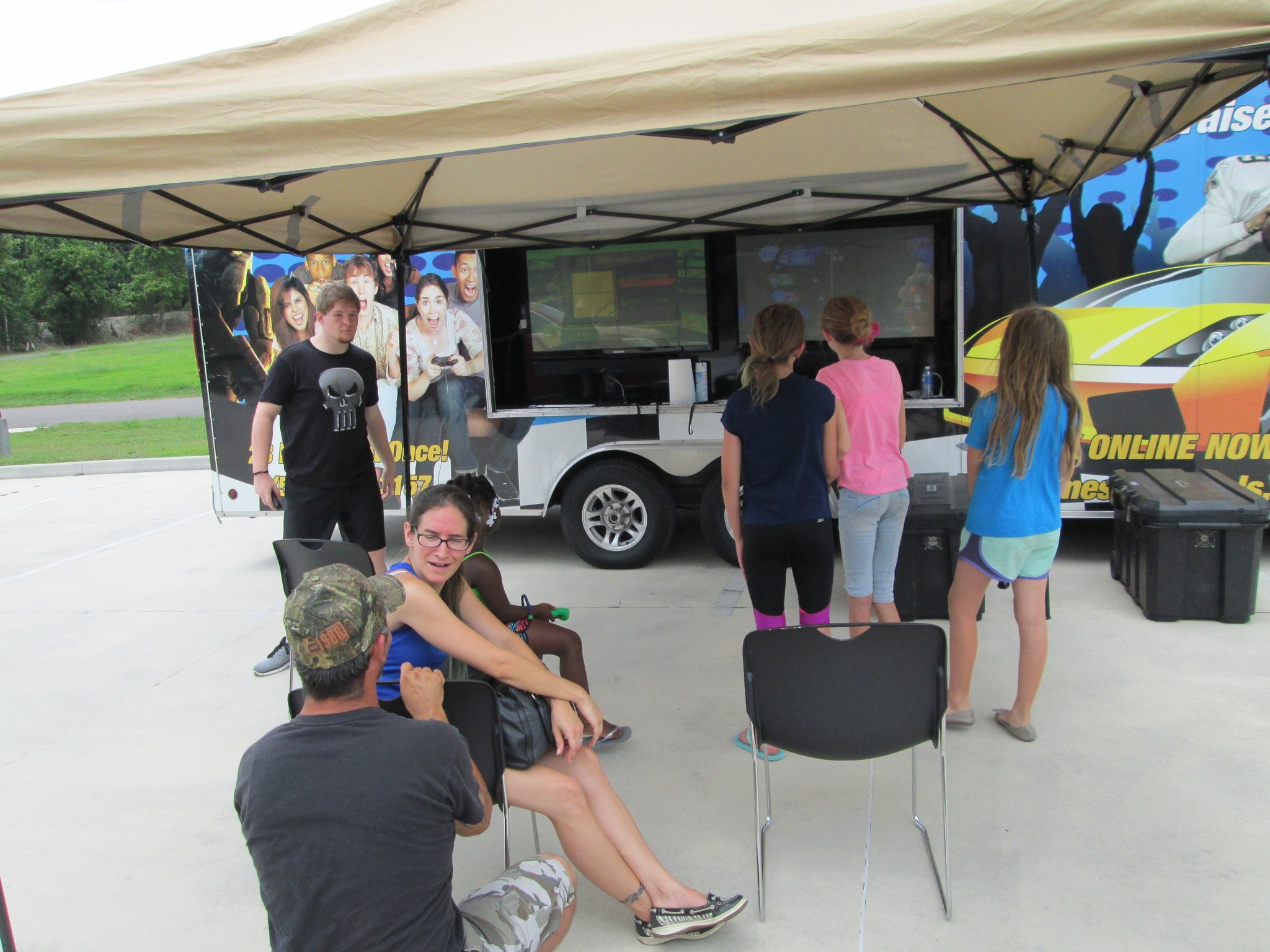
[{"xmin": 253, "ymin": 636, "xmax": 290, "ymax": 675}]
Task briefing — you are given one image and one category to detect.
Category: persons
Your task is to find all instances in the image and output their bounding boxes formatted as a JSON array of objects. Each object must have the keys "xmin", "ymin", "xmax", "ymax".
[
  {"xmin": 193, "ymin": 242, "xmax": 538, "ymax": 512},
  {"xmin": 373, "ymin": 484, "xmax": 748, "ymax": 945},
  {"xmin": 234, "ymin": 563, "xmax": 578, "ymax": 952},
  {"xmin": 764, "ymin": 245, "xmax": 813, "ymax": 319},
  {"xmin": 943, "ymin": 304, "xmax": 1085, "ymax": 741},
  {"xmin": 720, "ymin": 304, "xmax": 843, "ymax": 762},
  {"xmin": 445, "ymin": 473, "xmax": 633, "ymax": 747},
  {"xmin": 813, "ymin": 295, "xmax": 911, "ymax": 638},
  {"xmin": 250, "ymin": 280, "xmax": 396, "ymax": 679},
  {"xmin": 963, "ymin": 152, "xmax": 1270, "ymax": 331},
  {"xmin": 898, "ymin": 261, "xmax": 934, "ymax": 335}
]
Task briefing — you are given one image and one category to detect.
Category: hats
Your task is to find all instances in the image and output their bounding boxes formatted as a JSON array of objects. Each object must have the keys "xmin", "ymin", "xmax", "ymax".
[{"xmin": 283, "ymin": 564, "xmax": 406, "ymax": 670}]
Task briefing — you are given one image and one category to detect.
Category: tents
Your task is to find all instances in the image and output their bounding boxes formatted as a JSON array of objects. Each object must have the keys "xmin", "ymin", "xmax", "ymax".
[{"xmin": 0, "ymin": 0, "xmax": 1270, "ymax": 952}]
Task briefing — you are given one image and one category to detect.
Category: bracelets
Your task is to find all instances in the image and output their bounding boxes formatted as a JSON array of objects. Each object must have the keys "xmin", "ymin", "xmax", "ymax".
[{"xmin": 253, "ymin": 470, "xmax": 268, "ymax": 475}]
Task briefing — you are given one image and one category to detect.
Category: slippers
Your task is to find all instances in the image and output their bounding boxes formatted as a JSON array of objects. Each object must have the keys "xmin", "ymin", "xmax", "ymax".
[
  {"xmin": 734, "ymin": 729, "xmax": 784, "ymax": 761},
  {"xmin": 582, "ymin": 727, "xmax": 632, "ymax": 747}
]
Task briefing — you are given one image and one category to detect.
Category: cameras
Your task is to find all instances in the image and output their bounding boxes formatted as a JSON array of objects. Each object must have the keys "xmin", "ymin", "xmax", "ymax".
[{"xmin": 432, "ymin": 355, "xmax": 458, "ymax": 367}]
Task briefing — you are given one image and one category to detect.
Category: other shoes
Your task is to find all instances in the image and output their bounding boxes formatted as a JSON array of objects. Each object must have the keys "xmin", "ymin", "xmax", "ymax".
[
  {"xmin": 946, "ymin": 706, "xmax": 974, "ymax": 725},
  {"xmin": 634, "ymin": 913, "xmax": 726, "ymax": 946},
  {"xmin": 651, "ymin": 891, "xmax": 748, "ymax": 938},
  {"xmin": 484, "ymin": 468, "xmax": 519, "ymax": 503},
  {"xmin": 992, "ymin": 707, "xmax": 1037, "ymax": 742}
]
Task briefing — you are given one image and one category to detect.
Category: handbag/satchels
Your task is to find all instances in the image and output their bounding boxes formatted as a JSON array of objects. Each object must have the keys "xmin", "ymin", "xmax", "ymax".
[{"xmin": 494, "ymin": 681, "xmax": 556, "ymax": 771}]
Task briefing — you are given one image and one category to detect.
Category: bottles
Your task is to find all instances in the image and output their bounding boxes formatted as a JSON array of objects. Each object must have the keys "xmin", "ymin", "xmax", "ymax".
[
  {"xmin": 695, "ymin": 362, "xmax": 708, "ymax": 402},
  {"xmin": 921, "ymin": 366, "xmax": 933, "ymax": 398}
]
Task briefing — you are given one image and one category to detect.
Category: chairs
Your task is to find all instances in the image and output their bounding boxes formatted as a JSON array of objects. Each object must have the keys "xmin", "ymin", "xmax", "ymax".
[
  {"xmin": 288, "ymin": 680, "xmax": 540, "ymax": 873},
  {"xmin": 743, "ymin": 623, "xmax": 952, "ymax": 921},
  {"xmin": 272, "ymin": 538, "xmax": 376, "ymax": 692}
]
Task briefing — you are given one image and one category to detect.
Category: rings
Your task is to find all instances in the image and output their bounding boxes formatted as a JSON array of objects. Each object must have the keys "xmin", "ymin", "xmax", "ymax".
[{"xmin": 580, "ymin": 739, "xmax": 583, "ymax": 741}]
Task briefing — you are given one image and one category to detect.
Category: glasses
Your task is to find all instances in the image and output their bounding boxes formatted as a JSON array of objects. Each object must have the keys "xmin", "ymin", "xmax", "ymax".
[{"xmin": 414, "ymin": 526, "xmax": 471, "ymax": 550}]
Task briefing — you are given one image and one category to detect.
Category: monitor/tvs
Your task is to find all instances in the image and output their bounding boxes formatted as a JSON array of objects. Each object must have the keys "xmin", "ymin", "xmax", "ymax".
[
  {"xmin": 521, "ymin": 233, "xmax": 717, "ymax": 359},
  {"xmin": 730, "ymin": 214, "xmax": 942, "ymax": 349}
]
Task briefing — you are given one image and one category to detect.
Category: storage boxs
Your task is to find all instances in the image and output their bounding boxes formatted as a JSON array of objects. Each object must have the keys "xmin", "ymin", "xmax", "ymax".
[
  {"xmin": 1104, "ymin": 468, "xmax": 1270, "ymax": 625},
  {"xmin": 893, "ymin": 473, "xmax": 985, "ymax": 622}
]
